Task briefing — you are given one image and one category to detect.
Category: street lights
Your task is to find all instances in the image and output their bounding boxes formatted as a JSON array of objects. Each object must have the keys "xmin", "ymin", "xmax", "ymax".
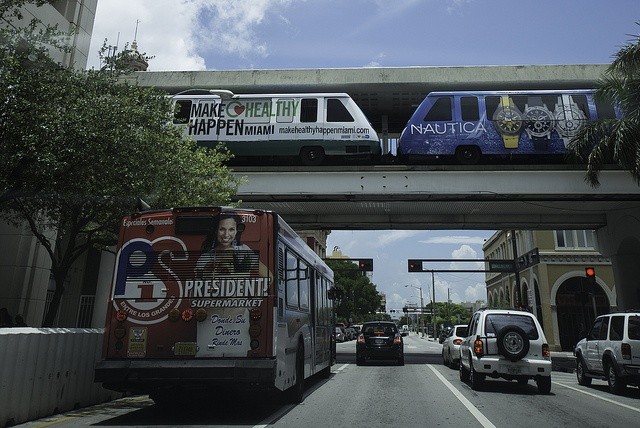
[
  {"xmin": 405, "ymin": 284, "xmax": 424, "ymax": 337},
  {"xmin": 170, "ymin": 88, "xmax": 234, "ymax": 100}
]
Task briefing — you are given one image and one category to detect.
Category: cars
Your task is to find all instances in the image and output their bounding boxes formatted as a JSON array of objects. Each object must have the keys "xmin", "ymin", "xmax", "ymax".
[
  {"xmin": 442, "ymin": 324, "xmax": 467, "ymax": 369},
  {"xmin": 335, "ymin": 327, "xmax": 344, "ymax": 342}
]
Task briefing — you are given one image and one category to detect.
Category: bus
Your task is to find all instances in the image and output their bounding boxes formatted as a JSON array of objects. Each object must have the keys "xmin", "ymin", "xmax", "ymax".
[{"xmin": 94, "ymin": 206, "xmax": 335, "ymax": 403}]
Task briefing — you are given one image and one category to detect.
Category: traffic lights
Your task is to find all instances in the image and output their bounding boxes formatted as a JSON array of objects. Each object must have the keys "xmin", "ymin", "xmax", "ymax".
[
  {"xmin": 407, "ymin": 259, "xmax": 422, "ymax": 271},
  {"xmin": 358, "ymin": 258, "xmax": 373, "ymax": 271},
  {"xmin": 585, "ymin": 267, "xmax": 594, "ymax": 277}
]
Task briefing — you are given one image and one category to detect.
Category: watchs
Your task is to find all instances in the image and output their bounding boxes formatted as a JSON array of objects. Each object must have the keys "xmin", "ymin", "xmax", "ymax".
[
  {"xmin": 492, "ymin": 93, "xmax": 523, "ymax": 148},
  {"xmin": 523, "ymin": 96, "xmax": 556, "ymax": 151},
  {"xmin": 552, "ymin": 93, "xmax": 590, "ymax": 151}
]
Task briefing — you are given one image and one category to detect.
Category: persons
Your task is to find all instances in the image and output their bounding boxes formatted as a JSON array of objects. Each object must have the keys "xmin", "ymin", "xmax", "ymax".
[{"xmin": 190, "ymin": 209, "xmax": 274, "ymax": 357}]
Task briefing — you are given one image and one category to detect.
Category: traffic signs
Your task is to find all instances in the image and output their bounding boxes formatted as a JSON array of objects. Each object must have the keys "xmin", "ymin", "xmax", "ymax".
[
  {"xmin": 489, "ymin": 260, "xmax": 515, "ymax": 271},
  {"xmin": 516, "ymin": 247, "xmax": 540, "ymax": 274}
]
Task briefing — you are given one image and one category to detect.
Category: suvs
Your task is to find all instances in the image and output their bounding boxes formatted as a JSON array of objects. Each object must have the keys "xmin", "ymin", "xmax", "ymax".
[
  {"xmin": 459, "ymin": 309, "xmax": 551, "ymax": 392},
  {"xmin": 356, "ymin": 321, "xmax": 409, "ymax": 366},
  {"xmin": 574, "ymin": 313, "xmax": 640, "ymax": 395}
]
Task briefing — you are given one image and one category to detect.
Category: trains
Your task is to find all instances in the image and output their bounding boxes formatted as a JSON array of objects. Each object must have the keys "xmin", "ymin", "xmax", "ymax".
[
  {"xmin": 397, "ymin": 89, "xmax": 624, "ymax": 165},
  {"xmin": 161, "ymin": 92, "xmax": 382, "ymax": 165}
]
